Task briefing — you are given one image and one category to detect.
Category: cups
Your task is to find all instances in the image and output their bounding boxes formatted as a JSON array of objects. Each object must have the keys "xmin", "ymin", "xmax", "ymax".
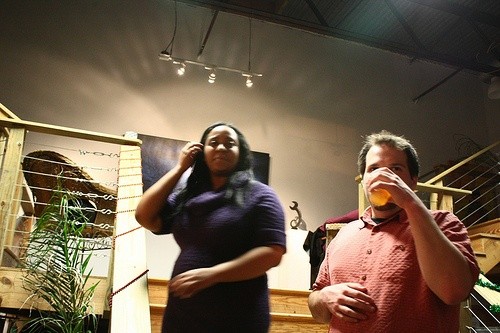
[{"xmin": 367, "ymin": 188, "xmax": 391, "ymax": 206}]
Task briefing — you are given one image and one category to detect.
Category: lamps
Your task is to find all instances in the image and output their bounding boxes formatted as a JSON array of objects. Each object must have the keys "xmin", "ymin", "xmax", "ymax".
[
  {"xmin": 480, "ymin": 69, "xmax": 500, "ymax": 100},
  {"xmin": 157, "ymin": 0, "xmax": 262, "ymax": 87}
]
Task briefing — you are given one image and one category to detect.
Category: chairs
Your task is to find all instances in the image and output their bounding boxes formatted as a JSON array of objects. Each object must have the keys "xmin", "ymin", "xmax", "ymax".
[{"xmin": 16, "ymin": 150, "xmax": 116, "ymax": 271}]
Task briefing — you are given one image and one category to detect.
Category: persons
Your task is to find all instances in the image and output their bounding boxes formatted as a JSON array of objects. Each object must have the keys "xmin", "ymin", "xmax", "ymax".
[
  {"xmin": 135, "ymin": 123, "xmax": 287, "ymax": 333},
  {"xmin": 307, "ymin": 130, "xmax": 480, "ymax": 333}
]
